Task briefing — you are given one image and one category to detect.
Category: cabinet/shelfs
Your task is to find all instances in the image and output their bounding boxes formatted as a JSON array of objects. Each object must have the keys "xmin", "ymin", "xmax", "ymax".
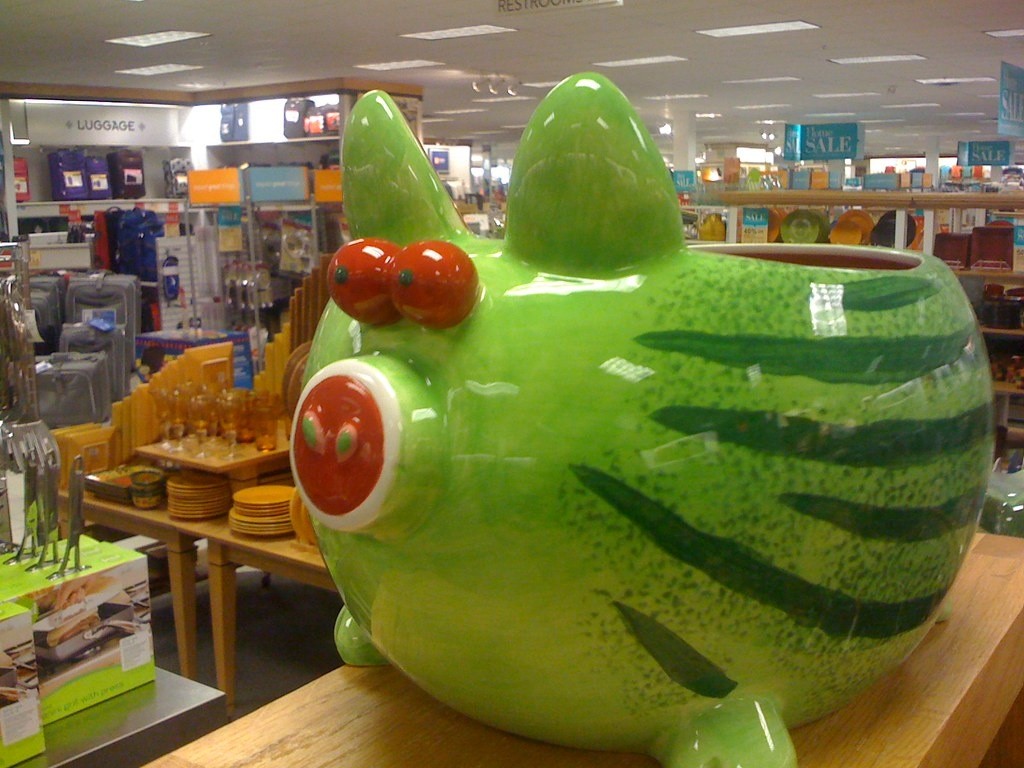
[
  {"xmin": 698, "ymin": 191, "xmax": 1024, "ymax": 440},
  {"xmin": 0, "ymin": 78, "xmax": 421, "ymax": 287}
]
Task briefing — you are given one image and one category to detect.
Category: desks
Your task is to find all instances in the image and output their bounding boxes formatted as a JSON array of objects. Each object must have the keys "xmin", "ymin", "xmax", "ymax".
[
  {"xmin": 57, "ymin": 491, "xmax": 336, "ymax": 720},
  {"xmin": 138, "ymin": 532, "xmax": 1024, "ymax": 768},
  {"xmin": 11, "ymin": 667, "xmax": 226, "ymax": 768}
]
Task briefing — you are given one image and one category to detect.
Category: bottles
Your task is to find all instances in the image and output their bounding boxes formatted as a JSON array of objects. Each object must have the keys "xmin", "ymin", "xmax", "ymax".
[{"xmin": 698, "ymin": 214, "xmax": 726, "ymax": 241}]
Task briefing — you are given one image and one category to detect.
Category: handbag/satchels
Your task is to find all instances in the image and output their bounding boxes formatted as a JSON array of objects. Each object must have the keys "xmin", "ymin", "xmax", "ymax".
[
  {"xmin": 113, "ymin": 206, "xmax": 193, "ymax": 282},
  {"xmin": 92, "ymin": 206, "xmax": 123, "ymax": 274}
]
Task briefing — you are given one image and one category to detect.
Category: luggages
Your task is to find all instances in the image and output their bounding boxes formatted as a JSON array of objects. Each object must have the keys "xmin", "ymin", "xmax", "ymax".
[
  {"xmin": 283, "ymin": 99, "xmax": 315, "ymax": 139},
  {"xmin": 12, "ymin": 155, "xmax": 30, "ymax": 203},
  {"xmin": 219, "ymin": 102, "xmax": 249, "ymax": 142},
  {"xmin": 85, "ymin": 155, "xmax": 112, "ymax": 200},
  {"xmin": 162, "ymin": 156, "xmax": 194, "ymax": 199},
  {"xmin": 47, "ymin": 147, "xmax": 88, "ymax": 201},
  {"xmin": 106, "ymin": 149, "xmax": 146, "ymax": 200},
  {"xmin": 303, "ymin": 102, "xmax": 341, "ymax": 137},
  {"xmin": 24, "ymin": 265, "xmax": 144, "ymax": 430}
]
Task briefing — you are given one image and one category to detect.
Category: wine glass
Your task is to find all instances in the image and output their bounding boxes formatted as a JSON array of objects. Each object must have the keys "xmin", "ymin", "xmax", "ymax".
[{"xmin": 154, "ymin": 378, "xmax": 245, "ymax": 460}]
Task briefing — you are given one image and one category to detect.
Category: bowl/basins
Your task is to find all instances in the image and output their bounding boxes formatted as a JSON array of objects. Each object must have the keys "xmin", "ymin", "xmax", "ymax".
[{"xmin": 129, "ymin": 470, "xmax": 162, "ymax": 510}]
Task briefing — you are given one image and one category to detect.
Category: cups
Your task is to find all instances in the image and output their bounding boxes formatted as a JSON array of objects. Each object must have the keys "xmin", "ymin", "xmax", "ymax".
[
  {"xmin": 207, "ymin": 381, "xmax": 297, "ymax": 451},
  {"xmin": 982, "ymin": 284, "xmax": 1024, "ymax": 330}
]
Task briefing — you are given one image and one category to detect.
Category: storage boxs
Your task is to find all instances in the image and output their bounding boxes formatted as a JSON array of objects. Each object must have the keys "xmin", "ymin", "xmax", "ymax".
[
  {"xmin": 135, "ymin": 327, "xmax": 253, "ymax": 388},
  {"xmin": 0, "ymin": 534, "xmax": 155, "ymax": 768}
]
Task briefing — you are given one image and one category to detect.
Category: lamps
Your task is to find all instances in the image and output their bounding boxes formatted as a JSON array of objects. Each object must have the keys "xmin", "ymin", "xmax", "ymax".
[
  {"xmin": 507, "ymin": 75, "xmax": 522, "ymax": 96},
  {"xmin": 488, "ymin": 72, "xmax": 505, "ymax": 94},
  {"xmin": 471, "ymin": 71, "xmax": 490, "ymax": 93}
]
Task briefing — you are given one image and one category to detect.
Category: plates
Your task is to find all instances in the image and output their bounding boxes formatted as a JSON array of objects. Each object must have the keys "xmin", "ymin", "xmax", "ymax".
[
  {"xmin": 281, "ymin": 342, "xmax": 313, "ymax": 420},
  {"xmin": 769, "ymin": 207, "xmax": 1013, "ymax": 271},
  {"xmin": 228, "ymin": 483, "xmax": 318, "ymax": 549},
  {"xmin": 84, "ymin": 461, "xmax": 168, "ymax": 503},
  {"xmin": 167, "ymin": 472, "xmax": 233, "ymax": 519}
]
